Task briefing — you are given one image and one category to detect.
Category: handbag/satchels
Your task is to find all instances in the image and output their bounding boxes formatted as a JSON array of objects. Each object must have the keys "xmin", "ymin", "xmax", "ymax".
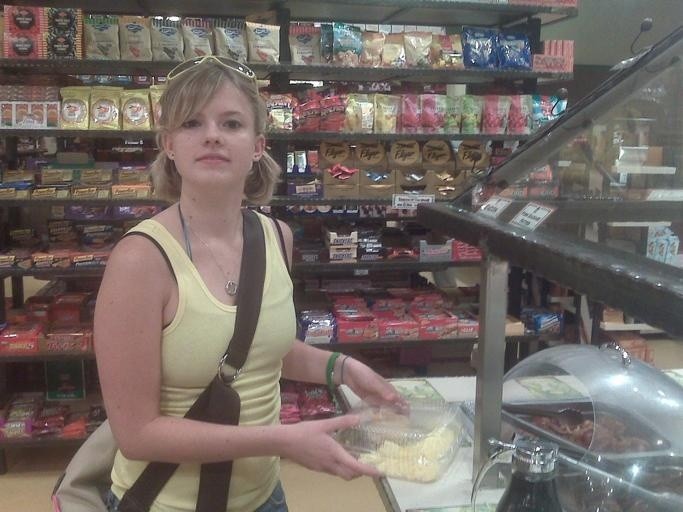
[{"xmin": 51, "ymin": 418, "xmax": 120, "ymax": 512}]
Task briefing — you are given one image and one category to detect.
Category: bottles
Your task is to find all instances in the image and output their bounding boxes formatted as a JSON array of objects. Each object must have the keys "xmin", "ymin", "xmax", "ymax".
[{"xmin": 494, "ymin": 439, "xmax": 563, "ymax": 511}]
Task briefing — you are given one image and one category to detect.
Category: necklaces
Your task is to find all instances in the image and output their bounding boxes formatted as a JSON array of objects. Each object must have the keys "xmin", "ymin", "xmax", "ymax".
[{"xmin": 183, "ymin": 214, "xmax": 240, "ymax": 296}]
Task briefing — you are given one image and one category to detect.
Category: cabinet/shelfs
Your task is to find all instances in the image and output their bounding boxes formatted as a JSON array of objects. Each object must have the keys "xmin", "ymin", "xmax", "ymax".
[{"xmin": 0, "ymin": 4, "xmax": 681, "ymax": 469}]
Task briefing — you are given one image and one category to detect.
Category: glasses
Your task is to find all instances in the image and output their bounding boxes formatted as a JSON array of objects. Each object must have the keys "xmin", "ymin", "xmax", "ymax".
[{"xmin": 166, "ymin": 56, "xmax": 259, "ymax": 95}]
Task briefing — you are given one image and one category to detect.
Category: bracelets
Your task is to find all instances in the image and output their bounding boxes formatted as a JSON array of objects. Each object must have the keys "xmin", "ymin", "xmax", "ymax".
[
  {"xmin": 326, "ymin": 351, "xmax": 341, "ymax": 391},
  {"xmin": 338, "ymin": 354, "xmax": 351, "ymax": 383}
]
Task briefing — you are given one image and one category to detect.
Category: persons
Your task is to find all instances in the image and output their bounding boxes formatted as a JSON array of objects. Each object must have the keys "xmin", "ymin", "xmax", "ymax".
[{"xmin": 91, "ymin": 54, "xmax": 411, "ymax": 512}]
